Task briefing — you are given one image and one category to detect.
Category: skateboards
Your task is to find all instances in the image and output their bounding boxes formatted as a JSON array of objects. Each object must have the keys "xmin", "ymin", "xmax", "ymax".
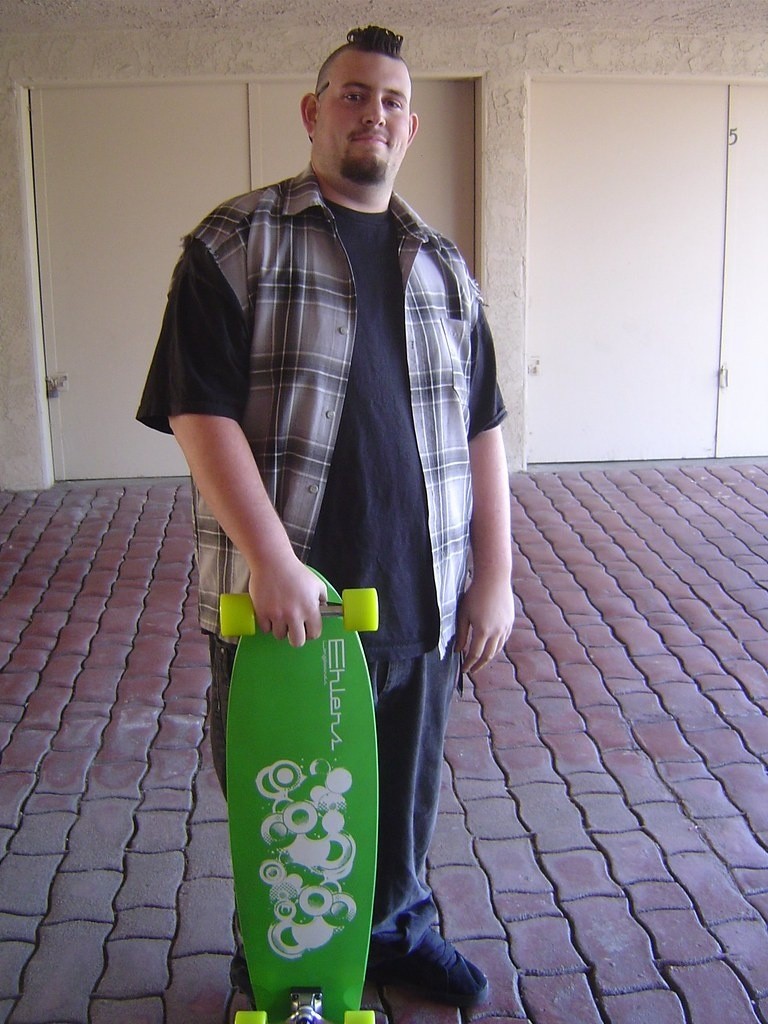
[{"xmin": 218, "ymin": 561, "xmax": 380, "ymax": 1024}]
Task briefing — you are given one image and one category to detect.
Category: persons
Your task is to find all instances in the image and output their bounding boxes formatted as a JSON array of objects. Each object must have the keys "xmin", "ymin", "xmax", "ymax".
[{"xmin": 136, "ymin": 25, "xmax": 514, "ymax": 1024}]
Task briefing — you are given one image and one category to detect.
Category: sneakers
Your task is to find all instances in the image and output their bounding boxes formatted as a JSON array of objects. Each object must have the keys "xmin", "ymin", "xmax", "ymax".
[
  {"xmin": 223, "ymin": 984, "xmax": 257, "ymax": 1024},
  {"xmin": 367, "ymin": 927, "xmax": 488, "ymax": 1006}
]
